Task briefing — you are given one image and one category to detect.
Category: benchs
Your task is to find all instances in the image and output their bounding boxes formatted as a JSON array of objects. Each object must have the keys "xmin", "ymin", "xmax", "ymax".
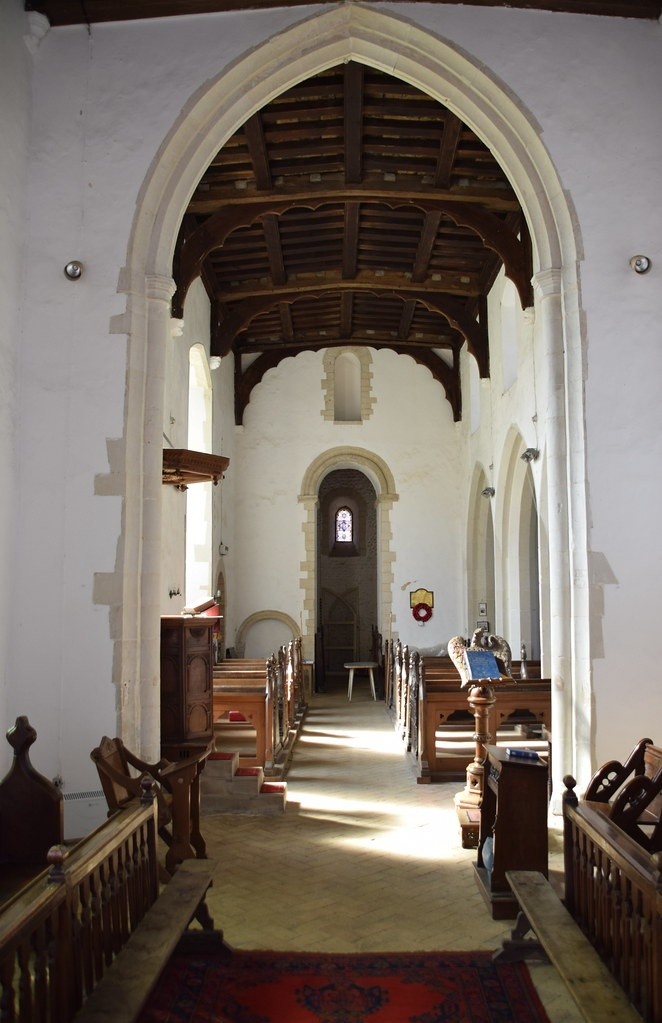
[
  {"xmin": 385, "ymin": 638, "xmax": 551, "ymax": 783},
  {"xmin": 73, "ymin": 858, "xmax": 219, "ymax": 1023},
  {"xmin": 213, "ymin": 636, "xmax": 302, "ymax": 777},
  {"xmin": 492, "ymin": 871, "xmax": 646, "ymax": 1023}
]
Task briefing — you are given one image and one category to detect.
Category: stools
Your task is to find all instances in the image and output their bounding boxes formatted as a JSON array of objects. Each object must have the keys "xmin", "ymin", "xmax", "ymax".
[{"xmin": 302, "ymin": 660, "xmax": 314, "ymax": 697}]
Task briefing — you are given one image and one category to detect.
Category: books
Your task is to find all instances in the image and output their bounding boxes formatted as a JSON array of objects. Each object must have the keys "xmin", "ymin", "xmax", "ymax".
[
  {"xmin": 464, "ymin": 650, "xmax": 500, "ymax": 680},
  {"xmin": 506, "ymin": 748, "xmax": 538, "ymax": 759}
]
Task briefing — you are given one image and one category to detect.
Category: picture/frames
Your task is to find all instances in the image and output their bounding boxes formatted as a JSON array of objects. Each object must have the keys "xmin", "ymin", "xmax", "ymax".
[
  {"xmin": 477, "ymin": 621, "xmax": 489, "ymax": 632},
  {"xmin": 479, "ymin": 603, "xmax": 487, "ymax": 615}
]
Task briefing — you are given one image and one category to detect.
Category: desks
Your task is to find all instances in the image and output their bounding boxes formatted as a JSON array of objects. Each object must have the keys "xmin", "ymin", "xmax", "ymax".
[{"xmin": 344, "ymin": 662, "xmax": 378, "ymax": 703}]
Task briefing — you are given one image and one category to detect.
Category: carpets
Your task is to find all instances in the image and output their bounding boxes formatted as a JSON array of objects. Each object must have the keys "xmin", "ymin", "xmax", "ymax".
[{"xmin": 139, "ymin": 945, "xmax": 551, "ymax": 1023}]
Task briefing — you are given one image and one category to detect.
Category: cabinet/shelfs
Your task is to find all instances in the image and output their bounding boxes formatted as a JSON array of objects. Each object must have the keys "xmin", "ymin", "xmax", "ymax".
[
  {"xmin": 473, "ymin": 745, "xmax": 548, "ymax": 921},
  {"xmin": 322, "ymin": 587, "xmax": 360, "ymax": 677},
  {"xmin": 161, "ymin": 615, "xmax": 223, "ymax": 775}
]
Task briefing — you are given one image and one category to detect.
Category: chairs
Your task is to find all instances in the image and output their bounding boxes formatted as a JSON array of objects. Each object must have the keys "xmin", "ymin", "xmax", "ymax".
[
  {"xmin": 90, "ymin": 735, "xmax": 212, "ymax": 875},
  {"xmin": 580, "ymin": 738, "xmax": 662, "ymax": 854}
]
